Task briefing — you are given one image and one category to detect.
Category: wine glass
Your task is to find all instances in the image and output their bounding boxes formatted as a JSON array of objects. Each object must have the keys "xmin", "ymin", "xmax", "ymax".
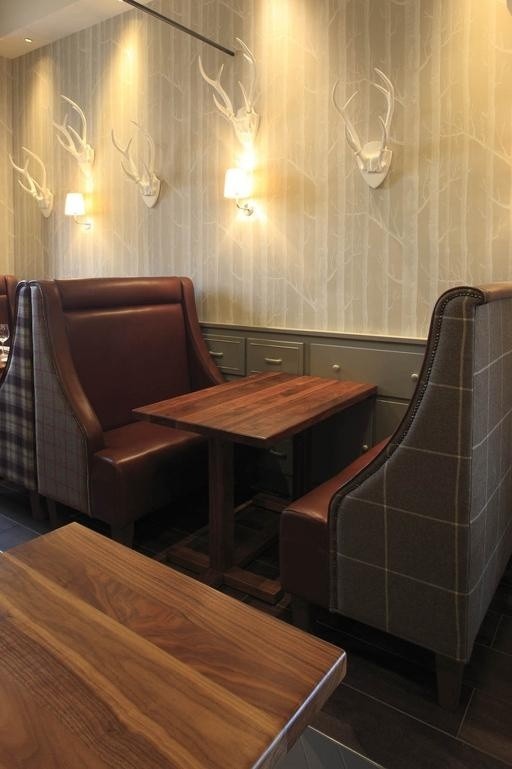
[{"xmin": 0, "ymin": 323, "xmax": 10, "ymax": 362}]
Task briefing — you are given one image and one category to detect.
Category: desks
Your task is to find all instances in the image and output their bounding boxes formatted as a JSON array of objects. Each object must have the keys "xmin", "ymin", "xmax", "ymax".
[{"xmin": 0, "ymin": 508, "xmax": 348, "ymax": 766}]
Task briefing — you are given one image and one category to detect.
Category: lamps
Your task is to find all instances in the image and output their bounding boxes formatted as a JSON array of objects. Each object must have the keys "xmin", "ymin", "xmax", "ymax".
[
  {"xmin": 223, "ymin": 168, "xmax": 256, "ymax": 217},
  {"xmin": 64, "ymin": 191, "xmax": 85, "ymax": 224}
]
